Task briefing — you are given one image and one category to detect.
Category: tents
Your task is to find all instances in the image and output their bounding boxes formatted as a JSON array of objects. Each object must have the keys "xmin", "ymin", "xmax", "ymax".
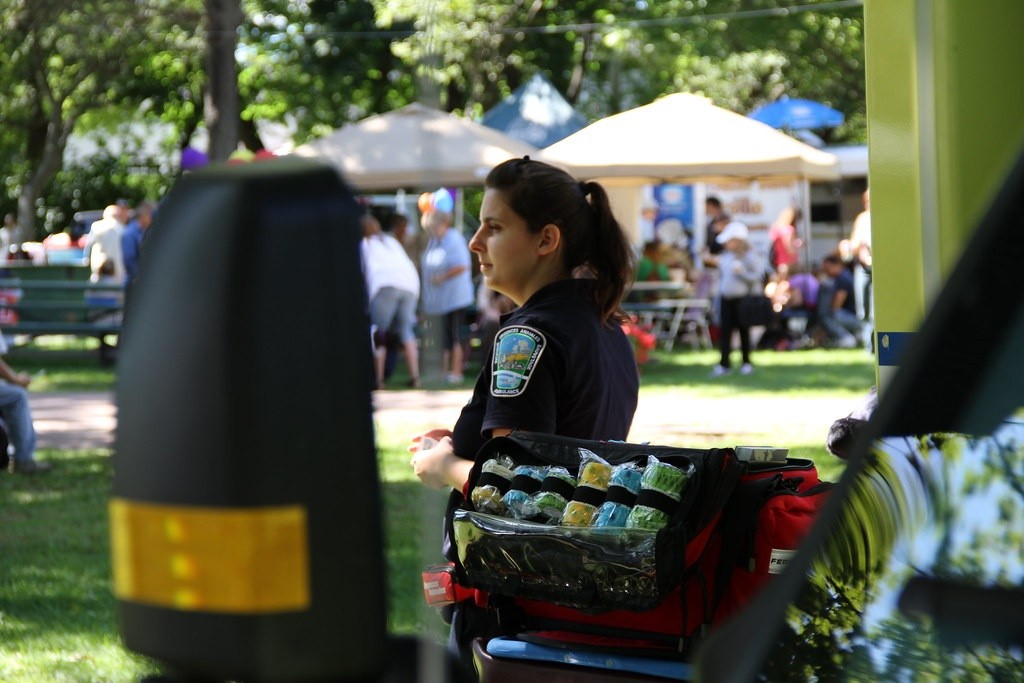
[
  {"xmin": 538, "ymin": 89, "xmax": 837, "ymax": 273},
  {"xmin": 283, "ymin": 101, "xmax": 540, "ymax": 188},
  {"xmin": 478, "ymin": 72, "xmax": 590, "ymax": 148}
]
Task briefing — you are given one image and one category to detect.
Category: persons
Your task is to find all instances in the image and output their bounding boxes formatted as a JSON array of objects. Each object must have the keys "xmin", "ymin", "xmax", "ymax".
[
  {"xmin": 418, "ymin": 210, "xmax": 475, "ymax": 383},
  {"xmin": 0, "ymin": 330, "xmax": 38, "ymax": 472},
  {"xmin": 477, "ymin": 279, "xmax": 515, "ymax": 369},
  {"xmin": 0, "ymin": 213, "xmax": 25, "ymax": 265},
  {"xmin": 40, "ymin": 199, "xmax": 155, "ymax": 325},
  {"xmin": 632, "ymin": 192, "xmax": 874, "ymax": 373},
  {"xmin": 360, "ymin": 215, "xmax": 421, "ymax": 390},
  {"xmin": 406, "ymin": 155, "xmax": 641, "ymax": 656}
]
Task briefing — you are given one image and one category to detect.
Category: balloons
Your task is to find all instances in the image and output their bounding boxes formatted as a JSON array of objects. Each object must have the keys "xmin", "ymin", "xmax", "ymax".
[{"xmin": 416, "ymin": 188, "xmax": 454, "ymax": 214}]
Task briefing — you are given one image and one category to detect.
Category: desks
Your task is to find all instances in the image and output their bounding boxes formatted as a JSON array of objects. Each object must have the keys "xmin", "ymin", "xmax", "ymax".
[{"xmin": 623, "ymin": 279, "xmax": 689, "ymax": 291}]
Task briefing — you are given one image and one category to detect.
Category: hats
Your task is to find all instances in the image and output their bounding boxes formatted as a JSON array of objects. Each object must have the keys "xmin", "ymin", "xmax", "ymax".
[{"xmin": 714, "ymin": 222, "xmax": 748, "ymax": 245}]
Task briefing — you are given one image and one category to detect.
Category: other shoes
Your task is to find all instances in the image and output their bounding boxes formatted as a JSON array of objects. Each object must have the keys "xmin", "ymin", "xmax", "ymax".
[
  {"xmin": 444, "ymin": 372, "xmax": 464, "ymax": 385},
  {"xmin": 7, "ymin": 459, "xmax": 51, "ymax": 477},
  {"xmin": 741, "ymin": 364, "xmax": 753, "ymax": 376},
  {"xmin": 708, "ymin": 366, "xmax": 731, "ymax": 379}
]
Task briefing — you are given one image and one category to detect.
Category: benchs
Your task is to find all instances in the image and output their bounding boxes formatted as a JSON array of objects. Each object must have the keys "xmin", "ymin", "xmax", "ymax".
[
  {"xmin": 619, "ymin": 298, "xmax": 711, "ymax": 352},
  {"xmin": 0, "ymin": 278, "xmax": 123, "ymax": 337}
]
